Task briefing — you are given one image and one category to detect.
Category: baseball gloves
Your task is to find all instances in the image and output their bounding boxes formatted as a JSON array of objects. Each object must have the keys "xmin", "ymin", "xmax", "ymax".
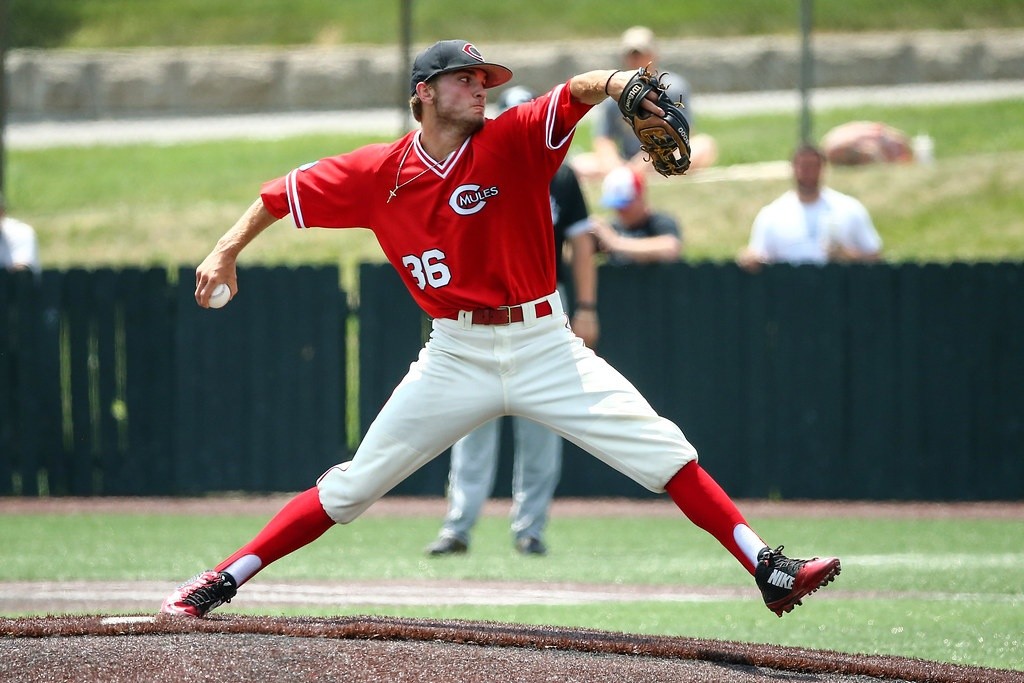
[{"xmin": 616, "ymin": 60, "xmax": 694, "ymax": 178}]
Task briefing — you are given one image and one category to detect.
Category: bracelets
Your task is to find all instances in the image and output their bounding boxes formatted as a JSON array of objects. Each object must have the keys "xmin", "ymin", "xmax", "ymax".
[{"xmin": 574, "ymin": 300, "xmax": 597, "ymax": 314}]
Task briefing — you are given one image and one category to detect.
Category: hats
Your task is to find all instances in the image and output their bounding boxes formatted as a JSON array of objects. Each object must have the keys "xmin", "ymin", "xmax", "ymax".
[
  {"xmin": 499, "ymin": 87, "xmax": 533, "ymax": 109},
  {"xmin": 599, "ymin": 167, "xmax": 649, "ymax": 209},
  {"xmin": 621, "ymin": 26, "xmax": 654, "ymax": 55},
  {"xmin": 412, "ymin": 40, "xmax": 513, "ymax": 94}
]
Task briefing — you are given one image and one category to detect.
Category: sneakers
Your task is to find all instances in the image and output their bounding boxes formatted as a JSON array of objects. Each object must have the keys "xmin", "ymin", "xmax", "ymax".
[
  {"xmin": 755, "ymin": 546, "xmax": 841, "ymax": 617},
  {"xmin": 161, "ymin": 571, "xmax": 237, "ymax": 617}
]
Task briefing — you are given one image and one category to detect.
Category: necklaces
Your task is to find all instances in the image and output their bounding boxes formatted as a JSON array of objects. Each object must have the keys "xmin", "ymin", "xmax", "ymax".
[{"xmin": 386, "ymin": 138, "xmax": 452, "ymax": 204}]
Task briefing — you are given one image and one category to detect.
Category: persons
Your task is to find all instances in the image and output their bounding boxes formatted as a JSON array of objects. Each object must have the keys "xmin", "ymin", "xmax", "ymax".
[
  {"xmin": 430, "ymin": 84, "xmax": 597, "ymax": 557},
  {"xmin": 162, "ymin": 40, "xmax": 842, "ymax": 619},
  {"xmin": 735, "ymin": 146, "xmax": 883, "ymax": 271},
  {"xmin": 594, "ymin": 26, "xmax": 718, "ymax": 172},
  {"xmin": 0, "ymin": 204, "xmax": 43, "ymax": 274},
  {"xmin": 590, "ymin": 165, "xmax": 685, "ymax": 265}
]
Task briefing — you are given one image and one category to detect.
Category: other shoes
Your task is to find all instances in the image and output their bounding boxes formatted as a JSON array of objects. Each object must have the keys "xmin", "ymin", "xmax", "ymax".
[
  {"xmin": 429, "ymin": 538, "xmax": 468, "ymax": 552},
  {"xmin": 516, "ymin": 538, "xmax": 545, "ymax": 551}
]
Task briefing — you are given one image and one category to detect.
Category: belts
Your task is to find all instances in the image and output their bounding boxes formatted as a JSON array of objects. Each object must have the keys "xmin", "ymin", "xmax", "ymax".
[{"xmin": 447, "ymin": 301, "xmax": 551, "ymax": 324}]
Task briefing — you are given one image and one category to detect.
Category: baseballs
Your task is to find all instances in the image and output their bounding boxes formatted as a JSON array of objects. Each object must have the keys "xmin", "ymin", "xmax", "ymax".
[{"xmin": 208, "ymin": 284, "xmax": 231, "ymax": 309}]
